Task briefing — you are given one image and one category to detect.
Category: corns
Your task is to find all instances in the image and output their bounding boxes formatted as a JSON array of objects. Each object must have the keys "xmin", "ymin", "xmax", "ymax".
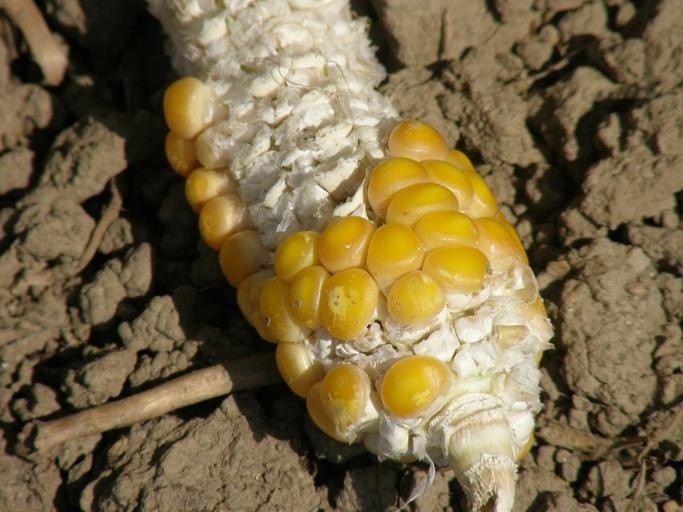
[{"xmin": 161, "ymin": 0, "xmax": 554, "ymax": 512}]
